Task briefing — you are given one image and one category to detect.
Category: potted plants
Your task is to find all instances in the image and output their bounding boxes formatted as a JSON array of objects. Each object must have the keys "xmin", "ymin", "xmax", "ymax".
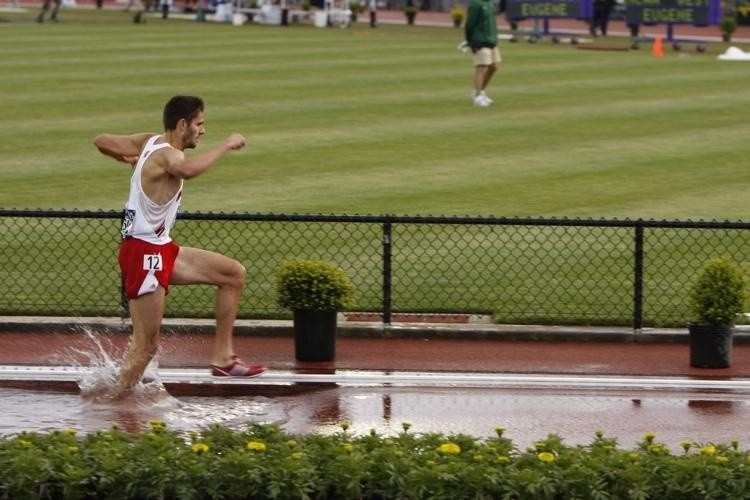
[
  {"xmin": 403, "ymin": 6, "xmax": 416, "ymax": 24},
  {"xmin": 278, "ymin": 259, "xmax": 353, "ymax": 361},
  {"xmin": 685, "ymin": 258, "xmax": 749, "ymax": 369},
  {"xmin": 451, "ymin": 11, "xmax": 465, "ymax": 30},
  {"xmin": 346, "ymin": 2, "xmax": 359, "ymax": 21}
]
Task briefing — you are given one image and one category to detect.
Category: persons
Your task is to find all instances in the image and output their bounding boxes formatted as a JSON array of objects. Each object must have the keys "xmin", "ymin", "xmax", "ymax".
[
  {"xmin": 626, "ymin": 0, "xmax": 645, "ymax": 38},
  {"xmin": 36, "ymin": 0, "xmax": 64, "ymax": 25},
  {"xmin": 463, "ymin": 0, "xmax": 502, "ymax": 110},
  {"xmin": 366, "ymin": 1, "xmax": 379, "ymax": 28},
  {"xmin": 161, "ymin": 0, "xmax": 173, "ymax": 20},
  {"xmin": 591, "ymin": 0, "xmax": 616, "ymax": 36},
  {"xmin": 280, "ymin": 1, "xmax": 293, "ymax": 26},
  {"xmin": 91, "ymin": 93, "xmax": 268, "ymax": 399},
  {"xmin": 132, "ymin": 0, "xmax": 146, "ymax": 25}
]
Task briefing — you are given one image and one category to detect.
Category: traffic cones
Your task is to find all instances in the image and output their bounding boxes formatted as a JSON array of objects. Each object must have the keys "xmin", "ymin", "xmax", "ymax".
[{"xmin": 652, "ymin": 33, "xmax": 665, "ymax": 57}]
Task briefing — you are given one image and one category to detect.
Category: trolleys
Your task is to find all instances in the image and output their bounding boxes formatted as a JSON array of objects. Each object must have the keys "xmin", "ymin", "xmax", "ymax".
[
  {"xmin": 625, "ymin": 0, "xmax": 722, "ymax": 53},
  {"xmin": 503, "ymin": 0, "xmax": 617, "ymax": 47}
]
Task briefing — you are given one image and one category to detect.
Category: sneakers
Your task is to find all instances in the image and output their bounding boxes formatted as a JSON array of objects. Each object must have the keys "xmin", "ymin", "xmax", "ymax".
[
  {"xmin": 209, "ymin": 353, "xmax": 269, "ymax": 378},
  {"xmin": 471, "ymin": 91, "xmax": 493, "ymax": 107}
]
